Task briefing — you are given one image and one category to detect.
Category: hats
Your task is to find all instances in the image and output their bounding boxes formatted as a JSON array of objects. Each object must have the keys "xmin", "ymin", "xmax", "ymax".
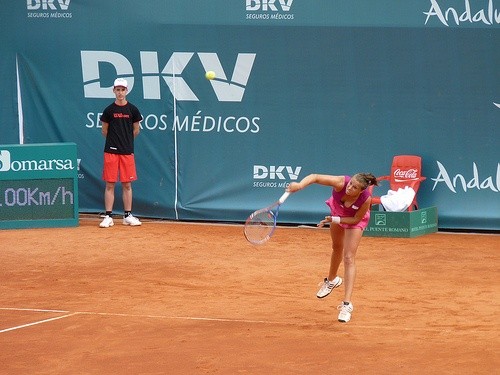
[{"xmin": 114, "ymin": 78, "xmax": 127, "ymax": 88}]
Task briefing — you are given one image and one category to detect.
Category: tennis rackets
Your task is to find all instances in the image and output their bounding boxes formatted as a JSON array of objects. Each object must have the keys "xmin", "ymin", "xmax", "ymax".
[{"xmin": 242, "ymin": 192, "xmax": 291, "ymax": 245}]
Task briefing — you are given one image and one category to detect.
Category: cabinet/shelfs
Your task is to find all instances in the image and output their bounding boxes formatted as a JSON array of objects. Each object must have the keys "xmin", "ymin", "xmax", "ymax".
[{"xmin": 0, "ymin": 143, "xmax": 78, "ymax": 229}]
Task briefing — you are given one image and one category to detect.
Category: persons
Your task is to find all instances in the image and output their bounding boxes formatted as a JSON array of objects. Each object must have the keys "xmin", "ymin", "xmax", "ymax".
[
  {"xmin": 284, "ymin": 172, "xmax": 380, "ymax": 323},
  {"xmin": 99, "ymin": 78, "xmax": 143, "ymax": 228}
]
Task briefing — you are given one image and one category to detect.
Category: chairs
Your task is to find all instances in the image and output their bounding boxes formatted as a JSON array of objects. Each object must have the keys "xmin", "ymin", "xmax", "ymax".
[{"xmin": 369, "ymin": 156, "xmax": 426, "ymax": 212}]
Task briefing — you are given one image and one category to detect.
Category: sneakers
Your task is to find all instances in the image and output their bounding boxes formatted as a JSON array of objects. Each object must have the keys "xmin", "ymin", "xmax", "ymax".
[
  {"xmin": 123, "ymin": 214, "xmax": 142, "ymax": 225},
  {"xmin": 337, "ymin": 301, "xmax": 353, "ymax": 322},
  {"xmin": 99, "ymin": 215, "xmax": 114, "ymax": 227},
  {"xmin": 316, "ymin": 276, "xmax": 342, "ymax": 298}
]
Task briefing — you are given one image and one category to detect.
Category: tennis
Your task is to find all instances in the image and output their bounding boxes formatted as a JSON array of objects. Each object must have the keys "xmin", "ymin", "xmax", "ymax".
[{"xmin": 204, "ymin": 71, "xmax": 216, "ymax": 80}]
